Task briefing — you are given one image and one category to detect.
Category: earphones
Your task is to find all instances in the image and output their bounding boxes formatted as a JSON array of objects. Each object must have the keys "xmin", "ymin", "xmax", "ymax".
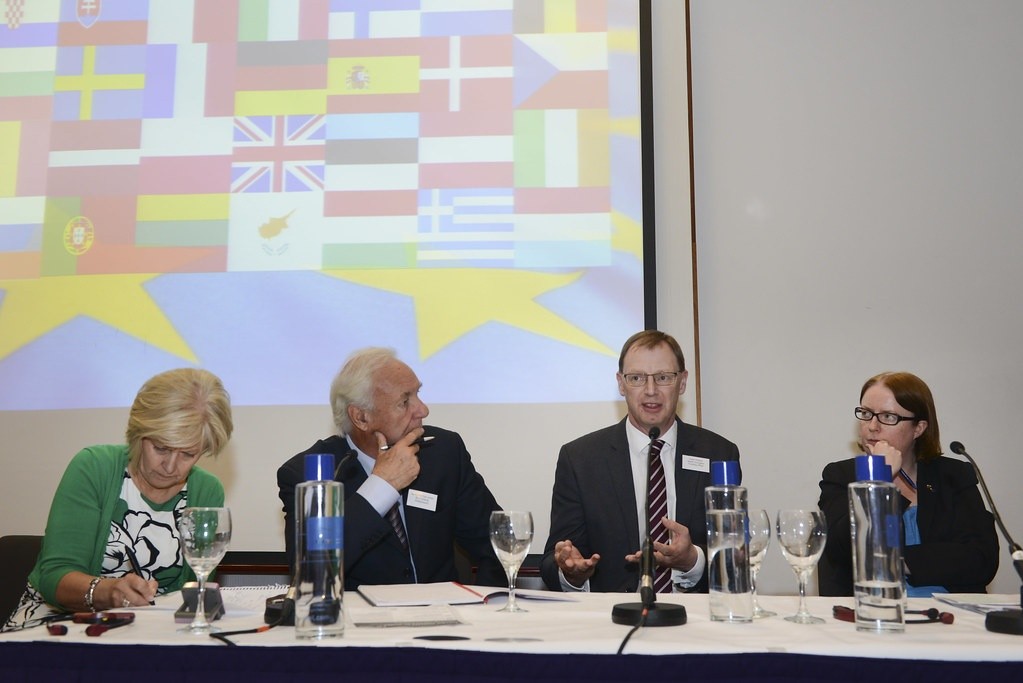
[
  {"xmin": 86, "ymin": 625, "xmax": 108, "ymax": 636},
  {"xmin": 939, "ymin": 612, "xmax": 954, "ymax": 624},
  {"xmin": 923, "ymin": 608, "xmax": 938, "ymax": 620},
  {"xmin": 48, "ymin": 626, "xmax": 67, "ymax": 635}
]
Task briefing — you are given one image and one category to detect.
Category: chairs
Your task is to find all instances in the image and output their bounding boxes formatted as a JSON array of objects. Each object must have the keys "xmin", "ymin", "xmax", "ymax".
[
  {"xmin": 214, "ymin": 564, "xmax": 291, "ymax": 589},
  {"xmin": 0, "ymin": 533, "xmax": 45, "ymax": 632},
  {"xmin": 472, "ymin": 565, "xmax": 551, "ymax": 591}
]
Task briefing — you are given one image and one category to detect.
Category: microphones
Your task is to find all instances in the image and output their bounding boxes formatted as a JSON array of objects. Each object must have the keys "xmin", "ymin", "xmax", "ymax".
[
  {"xmin": 950, "ymin": 441, "xmax": 1023, "ymax": 635},
  {"xmin": 264, "ymin": 449, "xmax": 358, "ymax": 625},
  {"xmin": 612, "ymin": 426, "xmax": 687, "ymax": 626}
]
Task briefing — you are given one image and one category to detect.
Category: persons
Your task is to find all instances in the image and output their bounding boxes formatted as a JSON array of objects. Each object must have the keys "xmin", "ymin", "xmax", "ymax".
[
  {"xmin": 815, "ymin": 373, "xmax": 999, "ymax": 597},
  {"xmin": 278, "ymin": 348, "xmax": 513, "ymax": 590},
  {"xmin": 0, "ymin": 368, "xmax": 233, "ymax": 633},
  {"xmin": 541, "ymin": 329, "xmax": 742, "ymax": 594}
]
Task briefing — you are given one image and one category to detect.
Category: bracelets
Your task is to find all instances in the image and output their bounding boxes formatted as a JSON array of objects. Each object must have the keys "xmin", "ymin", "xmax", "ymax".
[{"xmin": 85, "ymin": 576, "xmax": 106, "ymax": 613}]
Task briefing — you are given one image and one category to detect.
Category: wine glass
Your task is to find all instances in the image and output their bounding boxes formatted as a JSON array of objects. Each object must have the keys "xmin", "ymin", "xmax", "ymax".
[
  {"xmin": 746, "ymin": 509, "xmax": 776, "ymax": 618},
  {"xmin": 182, "ymin": 508, "xmax": 232, "ymax": 633},
  {"xmin": 489, "ymin": 510, "xmax": 534, "ymax": 613},
  {"xmin": 777, "ymin": 509, "xmax": 827, "ymax": 623}
]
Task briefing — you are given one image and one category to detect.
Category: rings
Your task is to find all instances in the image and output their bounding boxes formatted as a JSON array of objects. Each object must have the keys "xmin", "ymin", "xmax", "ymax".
[{"xmin": 123, "ymin": 599, "xmax": 130, "ymax": 608}]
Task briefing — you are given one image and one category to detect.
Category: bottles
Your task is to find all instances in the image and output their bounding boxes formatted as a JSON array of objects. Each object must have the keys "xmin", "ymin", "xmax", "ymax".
[
  {"xmin": 849, "ymin": 455, "xmax": 908, "ymax": 631},
  {"xmin": 294, "ymin": 454, "xmax": 344, "ymax": 638},
  {"xmin": 705, "ymin": 460, "xmax": 753, "ymax": 623}
]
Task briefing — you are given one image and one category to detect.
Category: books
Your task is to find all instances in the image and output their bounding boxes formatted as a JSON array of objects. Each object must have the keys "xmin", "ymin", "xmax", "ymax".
[
  {"xmin": 114, "ymin": 585, "xmax": 290, "ymax": 610},
  {"xmin": 350, "ymin": 605, "xmax": 466, "ymax": 626},
  {"xmin": 358, "ymin": 581, "xmax": 557, "ymax": 607},
  {"xmin": 930, "ymin": 593, "xmax": 1023, "ymax": 615}
]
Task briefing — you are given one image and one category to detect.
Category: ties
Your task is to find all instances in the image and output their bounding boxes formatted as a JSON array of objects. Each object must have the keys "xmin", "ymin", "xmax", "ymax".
[
  {"xmin": 385, "ymin": 502, "xmax": 410, "ymax": 559},
  {"xmin": 648, "ymin": 440, "xmax": 672, "ymax": 593}
]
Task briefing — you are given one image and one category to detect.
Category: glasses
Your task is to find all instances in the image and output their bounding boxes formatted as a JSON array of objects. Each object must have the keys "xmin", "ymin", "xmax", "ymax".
[
  {"xmin": 623, "ymin": 371, "xmax": 681, "ymax": 387},
  {"xmin": 855, "ymin": 407, "xmax": 914, "ymax": 425}
]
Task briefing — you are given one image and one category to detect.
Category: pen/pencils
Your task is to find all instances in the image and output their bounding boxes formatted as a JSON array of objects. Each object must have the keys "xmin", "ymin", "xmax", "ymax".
[
  {"xmin": 900, "ymin": 467, "xmax": 917, "ymax": 489},
  {"xmin": 381, "ymin": 435, "xmax": 435, "ymax": 449},
  {"xmin": 123, "ymin": 544, "xmax": 157, "ymax": 607}
]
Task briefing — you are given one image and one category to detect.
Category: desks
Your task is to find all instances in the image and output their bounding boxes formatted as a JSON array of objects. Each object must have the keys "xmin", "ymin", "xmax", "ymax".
[{"xmin": 0, "ymin": 589, "xmax": 1023, "ymax": 683}]
